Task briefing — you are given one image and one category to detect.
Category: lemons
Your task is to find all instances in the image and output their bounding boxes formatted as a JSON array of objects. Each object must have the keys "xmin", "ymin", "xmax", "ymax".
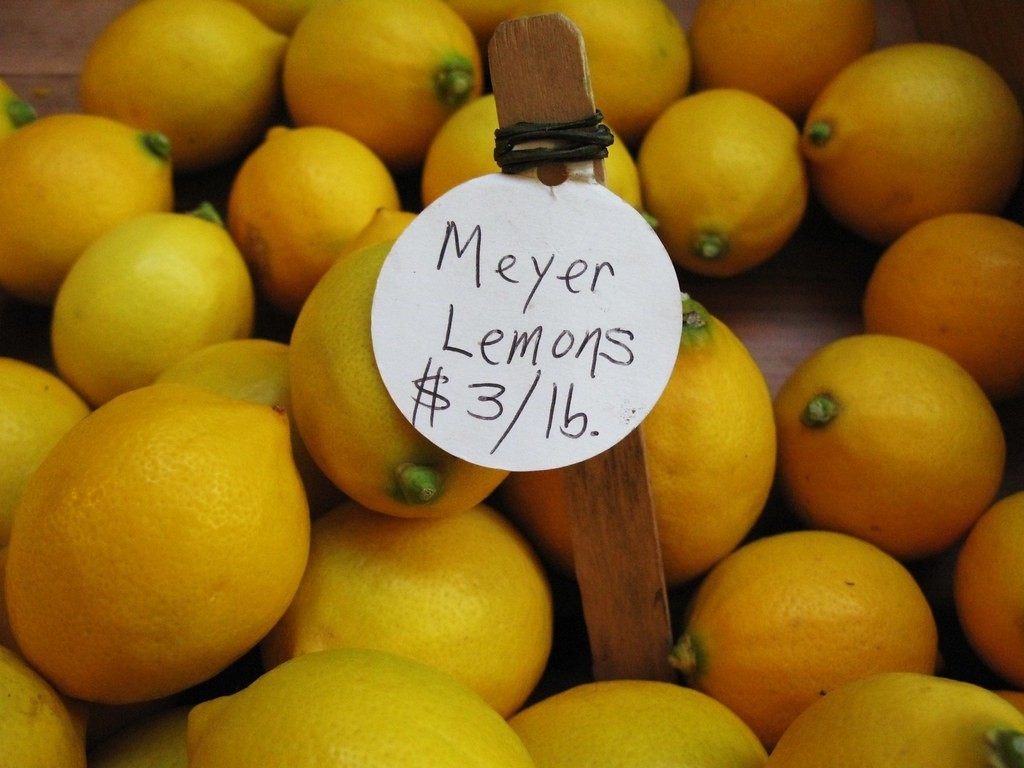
[{"xmin": 1, "ymin": 0, "xmax": 1024, "ymax": 768}]
